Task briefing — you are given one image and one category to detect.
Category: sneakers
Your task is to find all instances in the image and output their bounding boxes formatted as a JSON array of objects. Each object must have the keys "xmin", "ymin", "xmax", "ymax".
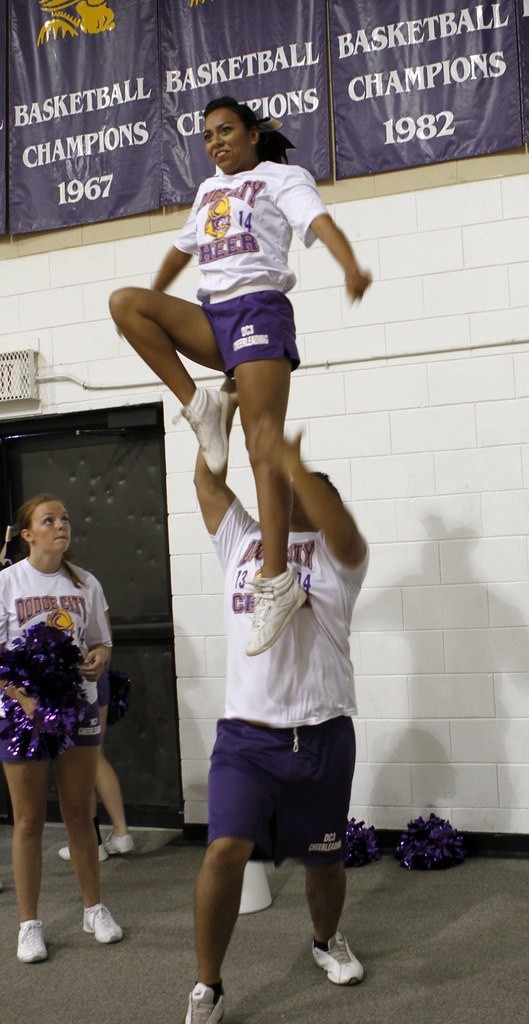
[
  {"xmin": 59, "ymin": 842, "xmax": 109, "ymax": 862},
  {"xmin": 173, "ymin": 388, "xmax": 230, "ymax": 475},
  {"xmin": 83, "ymin": 903, "xmax": 123, "ymax": 944},
  {"xmin": 104, "ymin": 831, "xmax": 135, "ymax": 854},
  {"xmin": 186, "ymin": 982, "xmax": 226, "ymax": 1024},
  {"xmin": 16, "ymin": 920, "xmax": 48, "ymax": 963},
  {"xmin": 242, "ymin": 562, "xmax": 309, "ymax": 656},
  {"xmin": 311, "ymin": 931, "xmax": 364, "ymax": 985}
]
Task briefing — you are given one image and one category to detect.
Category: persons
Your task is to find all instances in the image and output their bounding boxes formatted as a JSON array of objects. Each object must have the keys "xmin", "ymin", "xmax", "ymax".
[
  {"xmin": 109, "ymin": 93, "xmax": 374, "ymax": 656},
  {"xmin": 184, "ymin": 376, "xmax": 365, "ymax": 1024},
  {"xmin": 1, "ymin": 495, "xmax": 133, "ymax": 962}
]
